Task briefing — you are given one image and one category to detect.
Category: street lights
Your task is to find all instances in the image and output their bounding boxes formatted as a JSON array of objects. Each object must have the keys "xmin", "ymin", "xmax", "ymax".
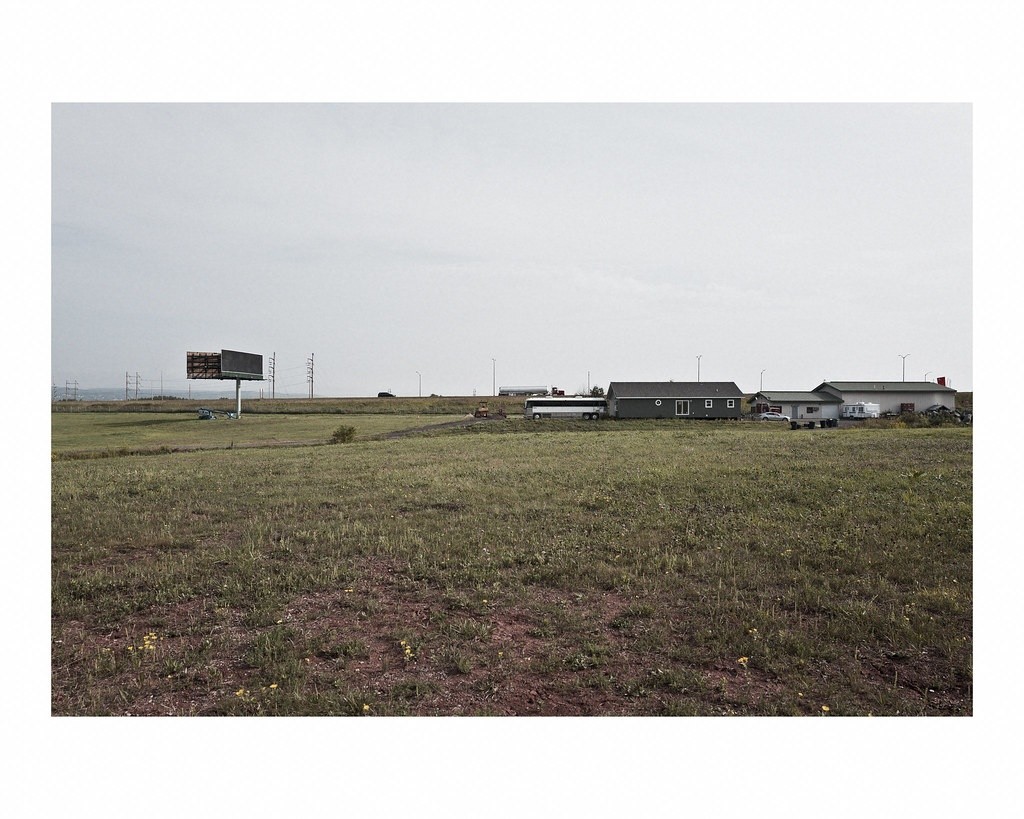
[
  {"xmin": 416, "ymin": 371, "xmax": 421, "ymax": 397},
  {"xmin": 899, "ymin": 354, "xmax": 910, "ymax": 382},
  {"xmin": 925, "ymin": 372, "xmax": 932, "ymax": 382},
  {"xmin": 760, "ymin": 370, "xmax": 765, "ymax": 391},
  {"xmin": 696, "ymin": 355, "xmax": 702, "ymax": 382}
]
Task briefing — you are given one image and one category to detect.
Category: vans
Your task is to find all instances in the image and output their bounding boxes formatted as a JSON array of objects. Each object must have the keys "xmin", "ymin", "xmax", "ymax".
[{"xmin": 378, "ymin": 392, "xmax": 396, "ymax": 397}]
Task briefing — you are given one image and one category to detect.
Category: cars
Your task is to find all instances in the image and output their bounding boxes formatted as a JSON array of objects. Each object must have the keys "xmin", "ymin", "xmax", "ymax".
[{"xmin": 759, "ymin": 412, "xmax": 790, "ymax": 423}]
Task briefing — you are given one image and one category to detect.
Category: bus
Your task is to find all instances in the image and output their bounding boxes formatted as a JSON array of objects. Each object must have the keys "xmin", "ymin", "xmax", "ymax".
[
  {"xmin": 842, "ymin": 403, "xmax": 881, "ymax": 420},
  {"xmin": 524, "ymin": 398, "xmax": 608, "ymax": 420}
]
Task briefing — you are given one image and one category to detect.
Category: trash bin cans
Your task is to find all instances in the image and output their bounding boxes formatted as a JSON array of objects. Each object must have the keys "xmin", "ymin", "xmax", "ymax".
[
  {"xmin": 820, "ymin": 420, "xmax": 826, "ymax": 428},
  {"xmin": 791, "ymin": 421, "xmax": 797, "ymax": 430},
  {"xmin": 826, "ymin": 418, "xmax": 837, "ymax": 428},
  {"xmin": 809, "ymin": 421, "xmax": 815, "ymax": 429}
]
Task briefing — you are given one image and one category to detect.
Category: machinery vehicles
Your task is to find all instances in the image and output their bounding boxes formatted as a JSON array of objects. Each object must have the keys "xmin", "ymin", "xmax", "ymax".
[{"xmin": 475, "ymin": 402, "xmax": 491, "ymax": 418}]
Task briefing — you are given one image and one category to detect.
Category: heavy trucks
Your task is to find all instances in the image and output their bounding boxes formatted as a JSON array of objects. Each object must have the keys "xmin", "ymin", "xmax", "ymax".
[{"xmin": 498, "ymin": 386, "xmax": 564, "ymax": 396}]
